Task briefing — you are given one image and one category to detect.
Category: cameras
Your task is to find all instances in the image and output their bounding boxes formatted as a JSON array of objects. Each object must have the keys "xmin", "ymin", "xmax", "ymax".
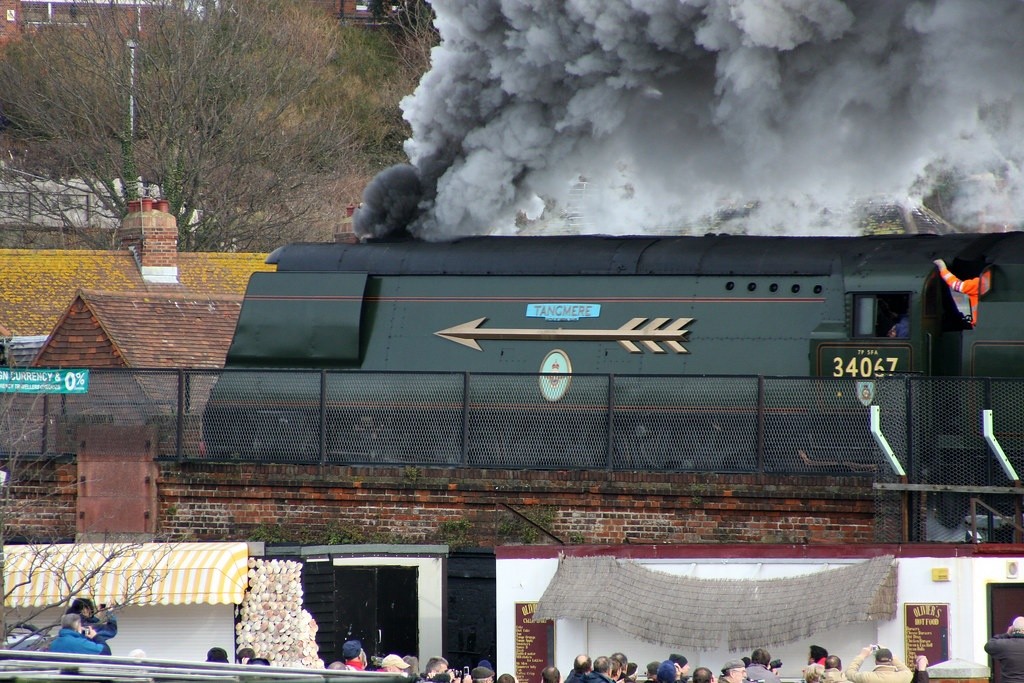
[
  {"xmin": 872, "ymin": 645, "xmax": 879, "ymax": 650},
  {"xmin": 100, "ymin": 604, "xmax": 106, "ymax": 609},
  {"xmin": 445, "ymin": 669, "xmax": 462, "ymax": 678},
  {"xmin": 769, "ymin": 659, "xmax": 782, "ymax": 673},
  {"xmin": 82, "ymin": 629, "xmax": 89, "ymax": 636}
]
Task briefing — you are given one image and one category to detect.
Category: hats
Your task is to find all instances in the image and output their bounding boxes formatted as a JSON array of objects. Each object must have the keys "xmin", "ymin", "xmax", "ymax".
[
  {"xmin": 875, "ymin": 649, "xmax": 893, "ymax": 662},
  {"xmin": 341, "ymin": 640, "xmax": 361, "ymax": 659},
  {"xmin": 721, "ymin": 659, "xmax": 745, "ymax": 671},
  {"xmin": 657, "ymin": 654, "xmax": 688, "ymax": 683},
  {"xmin": 381, "ymin": 654, "xmax": 411, "ymax": 670},
  {"xmin": 471, "ymin": 660, "xmax": 494, "ymax": 678}
]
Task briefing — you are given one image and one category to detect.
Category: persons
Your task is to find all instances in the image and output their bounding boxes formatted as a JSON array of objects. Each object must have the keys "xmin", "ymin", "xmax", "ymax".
[
  {"xmin": 878, "ymin": 303, "xmax": 913, "ymax": 338},
  {"xmin": 48, "ymin": 598, "xmax": 146, "ymax": 658},
  {"xmin": 933, "ymin": 259, "xmax": 992, "ymax": 330},
  {"xmin": 206, "ymin": 647, "xmax": 270, "ymax": 667},
  {"xmin": 984, "ymin": 616, "xmax": 1024, "ymax": 683},
  {"xmin": 327, "ymin": 639, "xmax": 782, "ymax": 683},
  {"xmin": 803, "ymin": 645, "xmax": 930, "ymax": 683}
]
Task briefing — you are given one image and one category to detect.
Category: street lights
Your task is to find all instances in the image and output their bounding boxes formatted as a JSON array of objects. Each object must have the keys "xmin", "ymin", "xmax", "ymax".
[{"xmin": 126, "ymin": 37, "xmax": 137, "ymax": 137}]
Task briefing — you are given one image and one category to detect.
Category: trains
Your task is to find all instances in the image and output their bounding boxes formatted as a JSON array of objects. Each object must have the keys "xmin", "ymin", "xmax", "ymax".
[{"xmin": 201, "ymin": 223, "xmax": 1024, "ymax": 541}]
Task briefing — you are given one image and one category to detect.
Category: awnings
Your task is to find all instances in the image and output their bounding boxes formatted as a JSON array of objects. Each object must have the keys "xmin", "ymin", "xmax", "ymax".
[{"xmin": 4, "ymin": 542, "xmax": 249, "ymax": 608}]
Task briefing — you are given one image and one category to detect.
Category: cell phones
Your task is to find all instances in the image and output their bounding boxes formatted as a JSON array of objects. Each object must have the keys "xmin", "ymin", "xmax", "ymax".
[{"xmin": 464, "ymin": 666, "xmax": 469, "ymax": 678}]
